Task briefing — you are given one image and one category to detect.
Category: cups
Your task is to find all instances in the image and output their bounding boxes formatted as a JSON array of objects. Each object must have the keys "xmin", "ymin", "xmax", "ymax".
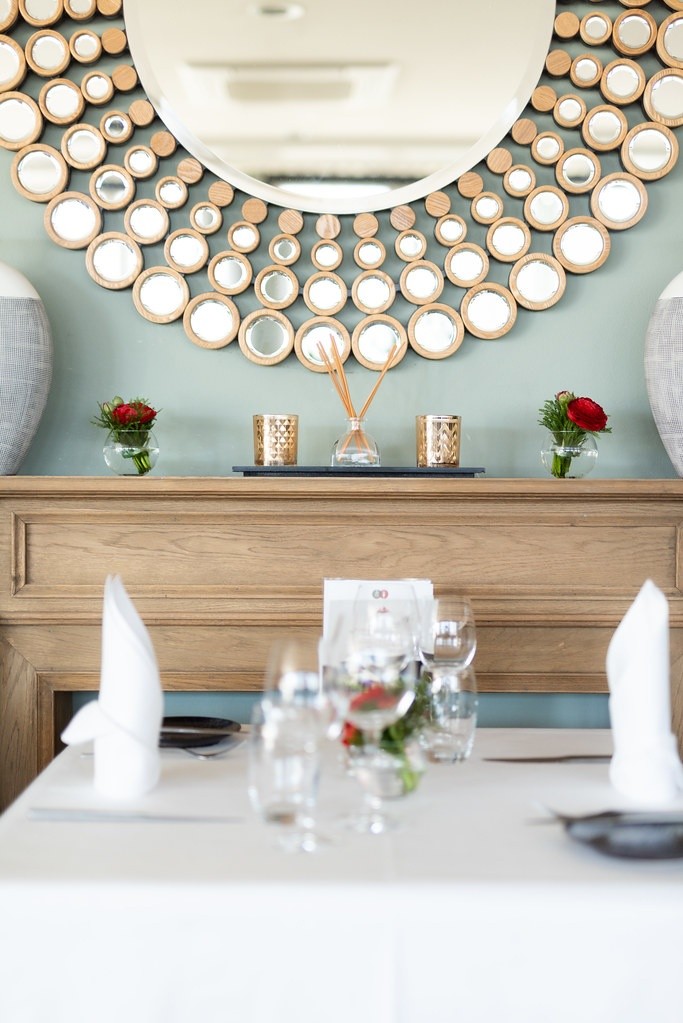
[
  {"xmin": 253, "ymin": 413, "xmax": 297, "ymax": 466},
  {"xmin": 415, "ymin": 414, "xmax": 462, "ymax": 469},
  {"xmin": 240, "ymin": 637, "xmax": 350, "ymax": 853},
  {"xmin": 415, "ymin": 663, "xmax": 478, "ymax": 763}
]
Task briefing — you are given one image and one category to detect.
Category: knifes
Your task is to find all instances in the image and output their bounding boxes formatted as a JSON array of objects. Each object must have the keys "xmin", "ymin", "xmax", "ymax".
[
  {"xmin": 482, "ymin": 753, "xmax": 612, "ymax": 767},
  {"xmin": 25, "ymin": 807, "xmax": 244, "ymax": 826}
]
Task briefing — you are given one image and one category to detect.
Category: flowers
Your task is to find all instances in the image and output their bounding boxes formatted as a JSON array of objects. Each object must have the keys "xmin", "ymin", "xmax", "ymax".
[
  {"xmin": 537, "ymin": 389, "xmax": 613, "ymax": 477},
  {"xmin": 88, "ymin": 396, "xmax": 163, "ymax": 473},
  {"xmin": 342, "ymin": 670, "xmax": 435, "ymax": 790}
]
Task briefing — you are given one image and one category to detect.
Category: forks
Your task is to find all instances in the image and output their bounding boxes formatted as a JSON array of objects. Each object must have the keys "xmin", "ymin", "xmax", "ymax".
[{"xmin": 81, "ymin": 740, "xmax": 248, "ymax": 762}]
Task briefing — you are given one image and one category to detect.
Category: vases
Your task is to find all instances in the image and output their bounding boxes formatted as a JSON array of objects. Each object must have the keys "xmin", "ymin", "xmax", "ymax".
[
  {"xmin": 348, "ymin": 715, "xmax": 432, "ymax": 797},
  {"xmin": 541, "ymin": 430, "xmax": 599, "ymax": 478},
  {"xmin": 103, "ymin": 429, "xmax": 160, "ymax": 477}
]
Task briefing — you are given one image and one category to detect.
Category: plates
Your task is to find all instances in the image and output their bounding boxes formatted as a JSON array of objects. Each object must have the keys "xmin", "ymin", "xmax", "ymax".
[{"xmin": 161, "ymin": 715, "xmax": 242, "ymax": 747}]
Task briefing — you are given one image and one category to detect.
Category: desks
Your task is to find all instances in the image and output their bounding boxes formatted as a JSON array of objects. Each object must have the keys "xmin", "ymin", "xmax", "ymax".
[
  {"xmin": 0, "ymin": 728, "xmax": 683, "ymax": 1023},
  {"xmin": 0, "ymin": 475, "xmax": 683, "ymax": 820}
]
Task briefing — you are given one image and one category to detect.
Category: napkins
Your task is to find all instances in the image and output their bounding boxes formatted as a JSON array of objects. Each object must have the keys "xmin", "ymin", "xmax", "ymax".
[
  {"xmin": 61, "ymin": 572, "xmax": 166, "ymax": 797},
  {"xmin": 603, "ymin": 575, "xmax": 683, "ymax": 812}
]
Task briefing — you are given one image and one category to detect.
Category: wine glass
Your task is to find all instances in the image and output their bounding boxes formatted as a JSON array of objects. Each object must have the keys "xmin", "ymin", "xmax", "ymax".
[
  {"xmin": 325, "ymin": 610, "xmax": 429, "ymax": 856},
  {"xmin": 413, "ymin": 594, "xmax": 478, "ymax": 752}
]
